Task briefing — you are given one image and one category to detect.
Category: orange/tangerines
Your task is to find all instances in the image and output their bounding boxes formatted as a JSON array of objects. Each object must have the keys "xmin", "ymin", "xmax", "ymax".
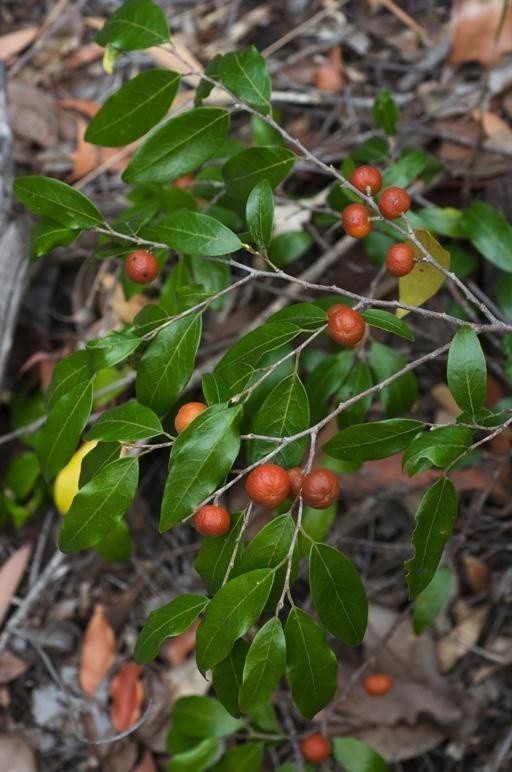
[
  {"xmin": 386, "ymin": 244, "xmax": 415, "ymax": 276},
  {"xmin": 245, "ymin": 464, "xmax": 341, "ymax": 509},
  {"xmin": 351, "ymin": 168, "xmax": 382, "ymax": 197},
  {"xmin": 342, "ymin": 204, "xmax": 374, "ymax": 239},
  {"xmin": 174, "ymin": 402, "xmax": 208, "ymax": 434},
  {"xmin": 194, "ymin": 506, "xmax": 231, "ymax": 536},
  {"xmin": 378, "ymin": 187, "xmax": 411, "ymax": 220},
  {"xmin": 364, "ymin": 673, "xmax": 392, "ymax": 695},
  {"xmin": 326, "ymin": 305, "xmax": 365, "ymax": 346},
  {"xmin": 301, "ymin": 734, "xmax": 330, "ymax": 762},
  {"xmin": 127, "ymin": 250, "xmax": 159, "ymax": 284}
]
